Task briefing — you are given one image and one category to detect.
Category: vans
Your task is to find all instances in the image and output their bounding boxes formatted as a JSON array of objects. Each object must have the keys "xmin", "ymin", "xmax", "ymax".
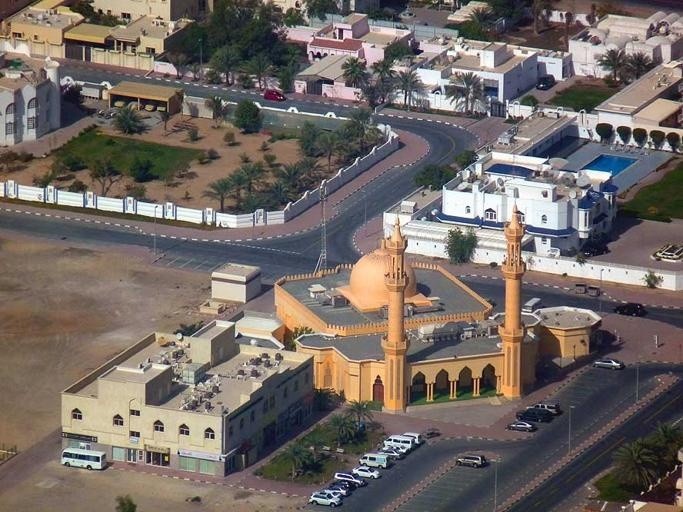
[{"xmin": 522, "ymin": 297, "xmax": 542, "ymax": 313}]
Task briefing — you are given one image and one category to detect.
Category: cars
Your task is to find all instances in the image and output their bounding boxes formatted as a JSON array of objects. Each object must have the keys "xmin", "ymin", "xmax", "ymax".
[
  {"xmin": 264, "ymin": 89, "xmax": 286, "ymax": 102},
  {"xmin": 614, "ymin": 302, "xmax": 647, "ymax": 317},
  {"xmin": 507, "ymin": 420, "xmax": 537, "ymax": 432},
  {"xmin": 308, "ymin": 431, "xmax": 422, "ymax": 508},
  {"xmin": 581, "ymin": 239, "xmax": 608, "ymax": 257},
  {"xmin": 536, "ymin": 75, "xmax": 555, "ymax": 91},
  {"xmin": 592, "ymin": 358, "xmax": 624, "ymax": 370}
]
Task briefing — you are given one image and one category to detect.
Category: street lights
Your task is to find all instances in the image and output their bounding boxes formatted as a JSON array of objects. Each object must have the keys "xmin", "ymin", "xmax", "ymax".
[
  {"xmin": 569, "ymin": 405, "xmax": 576, "ymax": 456},
  {"xmin": 636, "ymin": 362, "xmax": 641, "ymax": 403},
  {"xmin": 154, "ymin": 205, "xmax": 159, "ymax": 255},
  {"xmin": 600, "ymin": 268, "xmax": 604, "ymax": 312},
  {"xmin": 494, "ymin": 456, "xmax": 503, "ymax": 511},
  {"xmin": 198, "ymin": 38, "xmax": 204, "ymax": 86},
  {"xmin": 360, "ymin": 190, "xmax": 367, "ymax": 227}
]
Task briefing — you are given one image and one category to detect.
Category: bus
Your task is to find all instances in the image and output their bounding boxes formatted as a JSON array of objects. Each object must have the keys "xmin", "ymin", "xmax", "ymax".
[{"xmin": 61, "ymin": 447, "xmax": 107, "ymax": 471}]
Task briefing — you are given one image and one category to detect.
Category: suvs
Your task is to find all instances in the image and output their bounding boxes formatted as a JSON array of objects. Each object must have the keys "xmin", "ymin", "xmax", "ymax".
[
  {"xmin": 516, "ymin": 401, "xmax": 561, "ymax": 422},
  {"xmin": 456, "ymin": 454, "xmax": 486, "ymax": 468}
]
